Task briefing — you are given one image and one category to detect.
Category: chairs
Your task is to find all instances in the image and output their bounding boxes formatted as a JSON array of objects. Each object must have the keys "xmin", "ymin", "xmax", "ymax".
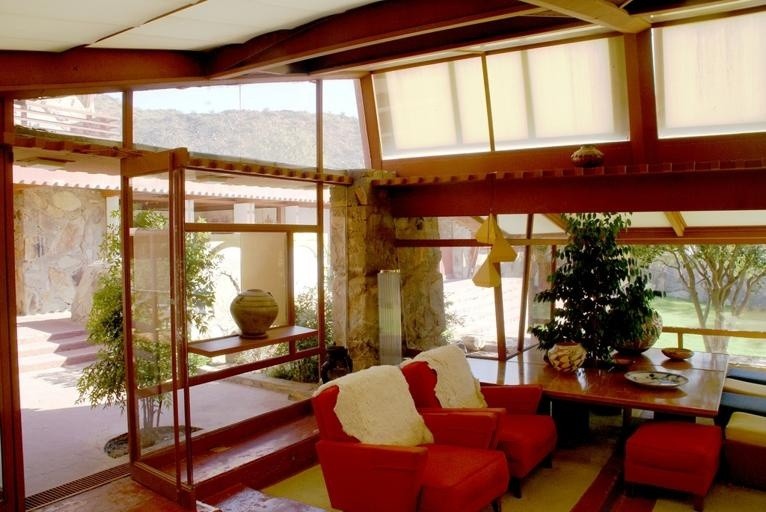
[
  {"xmin": 399, "ymin": 345, "xmax": 557, "ymax": 499},
  {"xmin": 313, "ymin": 364, "xmax": 510, "ymax": 511}
]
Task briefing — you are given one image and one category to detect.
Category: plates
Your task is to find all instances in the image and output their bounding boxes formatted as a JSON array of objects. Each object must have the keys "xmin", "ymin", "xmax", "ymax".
[{"xmin": 624, "ymin": 370, "xmax": 689, "ymax": 389}]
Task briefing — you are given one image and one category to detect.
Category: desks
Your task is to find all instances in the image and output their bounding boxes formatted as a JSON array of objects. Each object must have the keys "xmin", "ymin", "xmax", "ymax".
[{"xmin": 463, "ymin": 339, "xmax": 730, "ymax": 449}]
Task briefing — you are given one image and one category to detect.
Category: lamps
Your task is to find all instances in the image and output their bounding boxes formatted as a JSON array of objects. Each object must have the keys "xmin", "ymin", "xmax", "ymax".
[{"xmin": 378, "ymin": 268, "xmax": 403, "ymax": 368}]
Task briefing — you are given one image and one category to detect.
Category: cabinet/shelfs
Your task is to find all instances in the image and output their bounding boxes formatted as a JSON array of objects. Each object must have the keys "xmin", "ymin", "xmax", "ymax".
[{"xmin": 120, "ymin": 150, "xmax": 324, "ymax": 510}]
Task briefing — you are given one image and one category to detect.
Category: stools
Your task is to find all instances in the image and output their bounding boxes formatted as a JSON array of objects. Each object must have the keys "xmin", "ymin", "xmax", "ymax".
[
  {"xmin": 624, "ymin": 422, "xmax": 723, "ymax": 511},
  {"xmin": 718, "ymin": 360, "xmax": 764, "ymax": 489}
]
